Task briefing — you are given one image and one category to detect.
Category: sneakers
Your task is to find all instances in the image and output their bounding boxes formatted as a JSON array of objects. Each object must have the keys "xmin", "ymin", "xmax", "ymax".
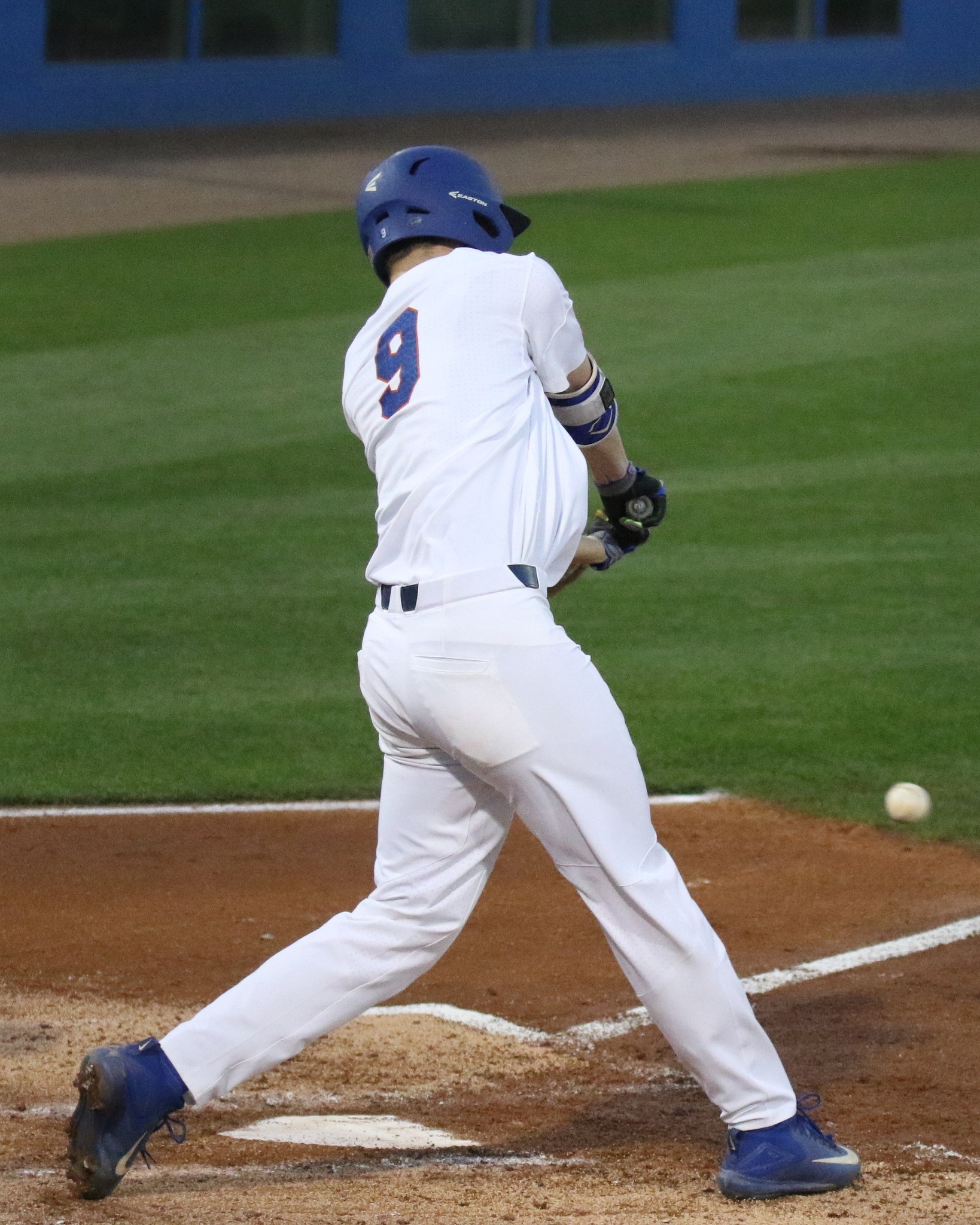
[
  {"xmin": 66, "ymin": 1037, "xmax": 189, "ymax": 1200},
  {"xmin": 717, "ymin": 1092, "xmax": 860, "ymax": 1200}
]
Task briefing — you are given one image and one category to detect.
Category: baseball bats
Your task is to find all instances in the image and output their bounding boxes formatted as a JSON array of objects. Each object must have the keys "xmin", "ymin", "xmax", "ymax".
[{"xmin": 546, "ymin": 495, "xmax": 655, "ymax": 599}]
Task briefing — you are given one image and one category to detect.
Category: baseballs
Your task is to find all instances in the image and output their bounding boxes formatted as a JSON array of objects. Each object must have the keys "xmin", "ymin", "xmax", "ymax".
[{"xmin": 882, "ymin": 782, "xmax": 934, "ymax": 821}]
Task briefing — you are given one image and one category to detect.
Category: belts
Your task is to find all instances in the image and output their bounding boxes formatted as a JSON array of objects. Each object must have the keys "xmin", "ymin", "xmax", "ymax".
[{"xmin": 378, "ymin": 565, "xmax": 539, "ymax": 611}]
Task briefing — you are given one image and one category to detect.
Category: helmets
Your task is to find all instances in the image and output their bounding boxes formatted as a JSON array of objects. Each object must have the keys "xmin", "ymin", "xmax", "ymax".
[{"xmin": 356, "ymin": 144, "xmax": 530, "ymax": 288}]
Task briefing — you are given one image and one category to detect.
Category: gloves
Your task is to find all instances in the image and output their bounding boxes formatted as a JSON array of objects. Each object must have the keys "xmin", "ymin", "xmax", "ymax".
[
  {"xmin": 601, "ymin": 468, "xmax": 667, "ymax": 530},
  {"xmin": 587, "ymin": 519, "xmax": 650, "ymax": 571}
]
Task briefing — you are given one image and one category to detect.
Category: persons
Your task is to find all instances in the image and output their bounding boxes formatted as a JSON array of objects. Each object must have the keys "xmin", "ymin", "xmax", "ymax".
[{"xmin": 63, "ymin": 146, "xmax": 862, "ymax": 1202}]
